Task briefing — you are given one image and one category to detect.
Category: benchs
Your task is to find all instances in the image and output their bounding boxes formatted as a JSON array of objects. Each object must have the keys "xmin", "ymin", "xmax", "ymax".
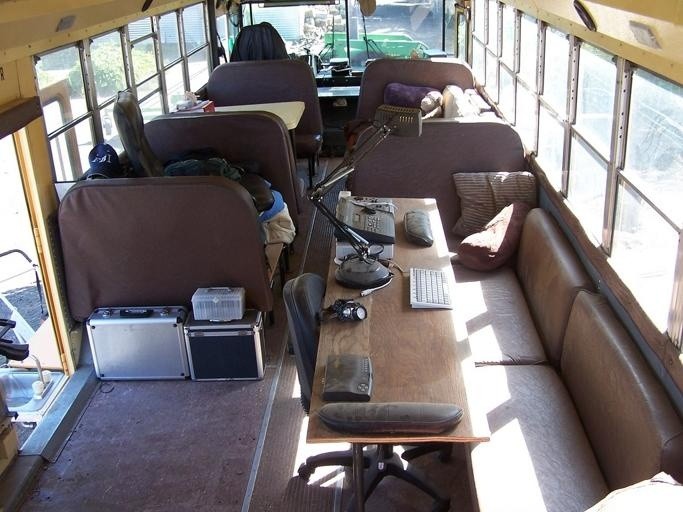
[
  {"xmin": 446, "ymin": 206, "xmax": 681, "ymax": 512},
  {"xmin": 58, "ymin": 59, "xmax": 324, "ymax": 324},
  {"xmin": 349, "ymin": 58, "xmax": 524, "ymax": 229}
]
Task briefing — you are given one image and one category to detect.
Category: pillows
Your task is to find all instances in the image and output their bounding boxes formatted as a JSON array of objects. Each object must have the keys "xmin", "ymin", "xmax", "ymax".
[{"xmin": 448, "ymin": 171, "xmax": 537, "ymax": 239}]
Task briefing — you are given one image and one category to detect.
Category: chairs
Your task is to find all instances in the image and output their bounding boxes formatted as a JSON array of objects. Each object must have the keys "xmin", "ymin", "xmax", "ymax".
[
  {"xmin": 281, "ymin": 272, "xmax": 456, "ymax": 512},
  {"xmin": 228, "ymin": 22, "xmax": 288, "ymax": 60}
]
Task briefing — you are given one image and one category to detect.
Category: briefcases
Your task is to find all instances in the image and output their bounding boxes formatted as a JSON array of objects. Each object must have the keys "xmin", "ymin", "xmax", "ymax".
[{"xmin": 85, "ymin": 304, "xmax": 192, "ymax": 381}]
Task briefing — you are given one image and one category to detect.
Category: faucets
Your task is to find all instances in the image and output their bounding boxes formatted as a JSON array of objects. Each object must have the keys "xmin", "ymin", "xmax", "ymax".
[{"xmin": 18, "ymin": 352, "xmax": 46, "ymax": 387}]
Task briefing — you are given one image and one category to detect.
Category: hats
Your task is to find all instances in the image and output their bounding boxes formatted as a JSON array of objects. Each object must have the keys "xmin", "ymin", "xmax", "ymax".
[{"xmin": 86, "ymin": 143, "xmax": 119, "ymax": 179}]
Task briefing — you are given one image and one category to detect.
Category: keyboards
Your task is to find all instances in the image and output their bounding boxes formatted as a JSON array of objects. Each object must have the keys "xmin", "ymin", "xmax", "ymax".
[{"xmin": 409, "ymin": 267, "xmax": 453, "ymax": 310}]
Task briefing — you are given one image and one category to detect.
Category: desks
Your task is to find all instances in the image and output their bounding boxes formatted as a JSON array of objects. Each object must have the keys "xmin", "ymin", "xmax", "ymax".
[{"xmin": 304, "ymin": 195, "xmax": 490, "ymax": 512}]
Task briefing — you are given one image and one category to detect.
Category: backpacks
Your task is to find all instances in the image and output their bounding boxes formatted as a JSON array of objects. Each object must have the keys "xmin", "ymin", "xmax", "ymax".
[{"xmin": 164, "ymin": 157, "xmax": 241, "ymax": 181}]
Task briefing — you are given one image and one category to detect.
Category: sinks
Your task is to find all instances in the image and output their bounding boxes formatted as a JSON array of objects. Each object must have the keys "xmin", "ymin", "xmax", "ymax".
[{"xmin": 1, "ymin": 370, "xmax": 53, "ymax": 410}]
